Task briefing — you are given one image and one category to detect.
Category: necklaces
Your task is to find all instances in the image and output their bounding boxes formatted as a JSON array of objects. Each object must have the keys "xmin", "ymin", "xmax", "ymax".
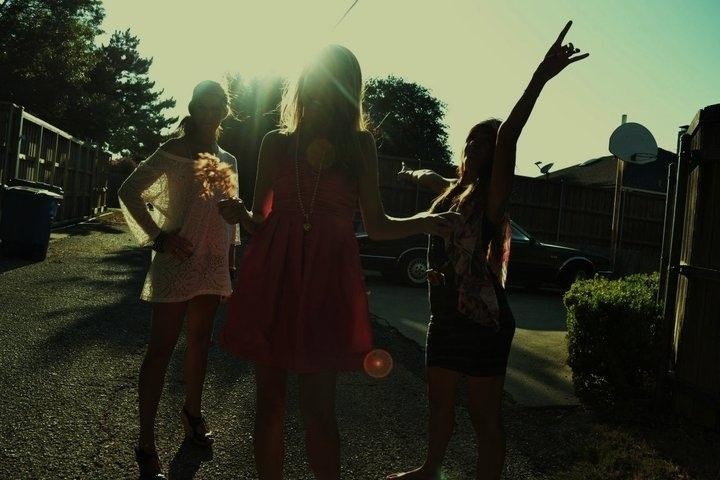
[{"xmin": 295, "ymin": 128, "xmax": 328, "ymax": 232}]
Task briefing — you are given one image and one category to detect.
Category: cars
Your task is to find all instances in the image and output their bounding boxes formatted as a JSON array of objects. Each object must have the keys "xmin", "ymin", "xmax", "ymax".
[{"xmin": 356, "ymin": 212, "xmax": 615, "ymax": 291}]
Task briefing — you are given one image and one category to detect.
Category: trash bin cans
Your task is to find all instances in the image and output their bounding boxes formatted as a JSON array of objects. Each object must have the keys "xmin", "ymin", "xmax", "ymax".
[{"xmin": 0, "ymin": 177, "xmax": 63, "ymax": 262}]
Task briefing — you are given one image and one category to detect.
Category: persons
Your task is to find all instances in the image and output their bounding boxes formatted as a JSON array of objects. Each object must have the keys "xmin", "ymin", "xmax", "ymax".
[
  {"xmin": 216, "ymin": 41, "xmax": 462, "ymax": 480},
  {"xmin": 117, "ymin": 80, "xmax": 243, "ymax": 480},
  {"xmin": 397, "ymin": 17, "xmax": 590, "ymax": 479}
]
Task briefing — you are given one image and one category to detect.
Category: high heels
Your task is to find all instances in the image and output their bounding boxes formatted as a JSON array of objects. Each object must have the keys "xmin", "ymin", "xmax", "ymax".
[
  {"xmin": 138, "ymin": 433, "xmax": 158, "ymax": 456},
  {"xmin": 179, "ymin": 406, "xmax": 214, "ymax": 447}
]
